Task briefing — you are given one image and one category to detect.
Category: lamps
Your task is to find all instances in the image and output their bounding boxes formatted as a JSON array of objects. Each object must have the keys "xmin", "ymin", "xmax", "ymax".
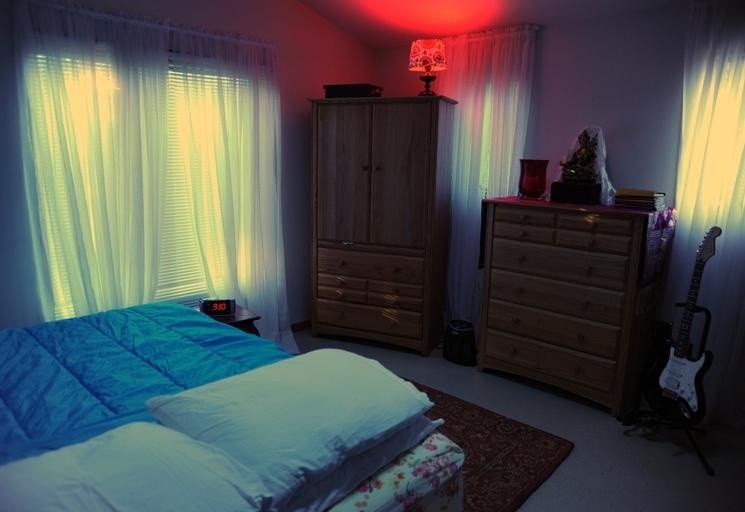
[{"xmin": 408, "ymin": 39, "xmax": 448, "ymax": 96}]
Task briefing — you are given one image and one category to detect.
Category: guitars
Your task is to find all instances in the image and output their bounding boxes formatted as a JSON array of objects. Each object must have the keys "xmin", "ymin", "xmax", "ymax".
[{"xmin": 646, "ymin": 226, "xmax": 721, "ymax": 427}]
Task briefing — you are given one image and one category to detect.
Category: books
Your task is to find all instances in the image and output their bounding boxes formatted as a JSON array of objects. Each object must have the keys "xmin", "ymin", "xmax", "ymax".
[{"xmin": 611, "ymin": 187, "xmax": 671, "ymax": 213}]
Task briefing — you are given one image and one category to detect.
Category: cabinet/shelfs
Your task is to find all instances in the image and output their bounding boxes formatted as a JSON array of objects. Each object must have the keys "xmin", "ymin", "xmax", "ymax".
[
  {"xmin": 306, "ymin": 97, "xmax": 459, "ymax": 357},
  {"xmin": 478, "ymin": 196, "xmax": 677, "ymax": 417}
]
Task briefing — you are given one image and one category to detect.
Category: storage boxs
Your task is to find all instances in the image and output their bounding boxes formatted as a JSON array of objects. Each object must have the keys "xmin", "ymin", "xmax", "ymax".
[{"xmin": 323, "ymin": 84, "xmax": 384, "ymax": 98}]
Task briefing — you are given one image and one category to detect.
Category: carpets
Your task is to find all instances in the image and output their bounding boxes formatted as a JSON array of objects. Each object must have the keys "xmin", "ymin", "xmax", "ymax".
[{"xmin": 402, "ymin": 378, "xmax": 575, "ymax": 512}]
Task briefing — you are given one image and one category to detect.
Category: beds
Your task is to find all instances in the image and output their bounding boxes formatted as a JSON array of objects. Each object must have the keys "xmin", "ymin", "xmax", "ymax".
[{"xmin": 1, "ymin": 304, "xmax": 466, "ymax": 512}]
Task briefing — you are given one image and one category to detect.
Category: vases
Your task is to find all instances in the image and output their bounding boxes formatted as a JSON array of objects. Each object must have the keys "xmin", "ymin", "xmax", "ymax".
[{"xmin": 519, "ymin": 159, "xmax": 549, "ymax": 200}]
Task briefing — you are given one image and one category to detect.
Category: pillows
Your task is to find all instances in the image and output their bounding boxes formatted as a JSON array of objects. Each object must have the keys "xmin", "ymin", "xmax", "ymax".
[
  {"xmin": 144, "ymin": 349, "xmax": 434, "ymax": 511},
  {"xmin": 2, "ymin": 422, "xmax": 275, "ymax": 512},
  {"xmin": 286, "ymin": 414, "xmax": 446, "ymax": 512}
]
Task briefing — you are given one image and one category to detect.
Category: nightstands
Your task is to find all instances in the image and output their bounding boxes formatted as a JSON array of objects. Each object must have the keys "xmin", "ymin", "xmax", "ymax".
[{"xmin": 190, "ymin": 304, "xmax": 261, "ymax": 336}]
